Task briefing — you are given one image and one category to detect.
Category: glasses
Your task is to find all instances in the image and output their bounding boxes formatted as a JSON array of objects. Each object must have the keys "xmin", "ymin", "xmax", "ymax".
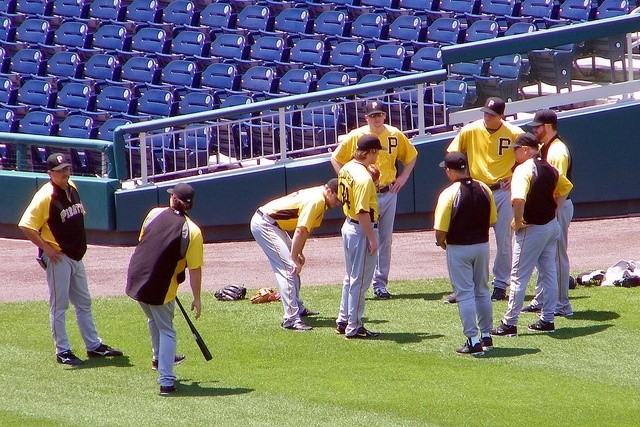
[{"xmin": 369, "ymin": 113, "xmax": 385, "ymax": 118}]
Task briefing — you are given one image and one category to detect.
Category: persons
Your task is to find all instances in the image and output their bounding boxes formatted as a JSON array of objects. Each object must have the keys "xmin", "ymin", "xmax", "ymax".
[
  {"xmin": 125, "ymin": 183, "xmax": 204, "ymax": 394},
  {"xmin": 488, "ymin": 132, "xmax": 574, "ymax": 336},
  {"xmin": 336, "ymin": 133, "xmax": 388, "ymax": 338},
  {"xmin": 433, "ymin": 151, "xmax": 501, "ymax": 356},
  {"xmin": 250, "ymin": 177, "xmax": 342, "ymax": 331},
  {"xmin": 525, "ymin": 109, "xmax": 575, "ymax": 318},
  {"xmin": 330, "ymin": 100, "xmax": 419, "ymax": 300},
  {"xmin": 445, "ymin": 96, "xmax": 524, "ymax": 303},
  {"xmin": 17, "ymin": 152, "xmax": 123, "ymax": 365}
]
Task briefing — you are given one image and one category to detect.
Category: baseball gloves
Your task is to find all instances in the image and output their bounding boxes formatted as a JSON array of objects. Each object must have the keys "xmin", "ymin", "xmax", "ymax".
[
  {"xmin": 251, "ymin": 288, "xmax": 281, "ymax": 304},
  {"xmin": 214, "ymin": 284, "xmax": 246, "ymax": 300}
]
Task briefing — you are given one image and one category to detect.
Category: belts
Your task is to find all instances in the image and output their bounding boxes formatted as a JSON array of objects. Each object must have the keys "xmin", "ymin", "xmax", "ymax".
[
  {"xmin": 256, "ymin": 208, "xmax": 282, "ymax": 229},
  {"xmin": 376, "ymin": 182, "xmax": 394, "ymax": 194},
  {"xmin": 351, "ymin": 220, "xmax": 380, "ymax": 228}
]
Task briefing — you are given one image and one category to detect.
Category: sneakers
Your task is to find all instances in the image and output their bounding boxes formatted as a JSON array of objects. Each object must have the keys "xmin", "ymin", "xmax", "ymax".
[
  {"xmin": 577, "ymin": 270, "xmax": 606, "ymax": 286},
  {"xmin": 87, "ymin": 344, "xmax": 113, "ymax": 356},
  {"xmin": 490, "ymin": 323, "xmax": 518, "ymax": 337},
  {"xmin": 456, "ymin": 341, "xmax": 485, "ymax": 356},
  {"xmin": 521, "ymin": 303, "xmax": 541, "ymax": 313},
  {"xmin": 286, "ymin": 321, "xmax": 312, "ymax": 330},
  {"xmin": 481, "ymin": 336, "xmax": 493, "ymax": 351},
  {"xmin": 491, "ymin": 287, "xmax": 507, "ymax": 301},
  {"xmin": 528, "ymin": 320, "xmax": 555, "ymax": 332},
  {"xmin": 302, "ymin": 309, "xmax": 319, "ymax": 316},
  {"xmin": 346, "ymin": 327, "xmax": 378, "ymax": 338},
  {"xmin": 158, "ymin": 385, "xmax": 178, "ymax": 395},
  {"xmin": 335, "ymin": 322, "xmax": 347, "ymax": 334},
  {"xmin": 373, "ymin": 287, "xmax": 392, "ymax": 299},
  {"xmin": 57, "ymin": 350, "xmax": 76, "ymax": 364},
  {"xmin": 152, "ymin": 355, "xmax": 186, "ymax": 371}
]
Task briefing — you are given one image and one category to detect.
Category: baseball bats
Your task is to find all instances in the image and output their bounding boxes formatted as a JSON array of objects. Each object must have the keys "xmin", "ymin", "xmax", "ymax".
[{"xmin": 175, "ymin": 296, "xmax": 213, "ymax": 361}]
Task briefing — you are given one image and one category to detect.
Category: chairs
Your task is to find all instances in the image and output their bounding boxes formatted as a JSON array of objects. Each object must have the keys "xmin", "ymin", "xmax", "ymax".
[
  {"xmin": 0, "ymin": 48, "xmax": 9, "ymax": 74},
  {"xmin": 245, "ymin": 98, "xmax": 296, "ymax": 157},
  {"xmin": 234, "ymin": 38, "xmax": 284, "ymax": 66},
  {"xmin": 393, "ymin": 48, "xmax": 447, "ymax": 75},
  {"xmin": 260, "ymin": 9, "xmax": 308, "ymax": 38},
  {"xmin": 210, "ymin": 34, "xmax": 251, "ymax": 63},
  {"xmin": 214, "ymin": 98, "xmax": 254, "ymax": 158},
  {"xmin": 594, "ymin": 0, "xmax": 631, "ymax": 83},
  {"xmin": 0, "ymin": 107, "xmax": 15, "ymax": 158},
  {"xmin": 346, "ymin": 2, "xmax": 392, "ymax": 15},
  {"xmin": 128, "ymin": 27, "xmax": 170, "ymax": 60},
  {"xmin": 0, "ymin": 49, "xmax": 41, "ymax": 82},
  {"xmin": 0, "ymin": 1, "xmax": 48, "ymax": 18},
  {"xmin": 125, "ymin": 125, "xmax": 174, "ymax": 175},
  {"xmin": 352, "ymin": 45, "xmax": 404, "ymax": 79},
  {"xmin": 121, "ymin": 89, "xmax": 173, "ymax": 120},
  {"xmin": 1, "ymin": 18, "xmax": 13, "ymax": 45},
  {"xmin": 221, "ymin": 6, "xmax": 270, "ymax": 35},
  {"xmin": 92, "ymin": 24, "xmax": 132, "ymax": 55},
  {"xmin": 0, "ymin": 75, "xmax": 13, "ymax": 109},
  {"xmin": 274, "ymin": 40, "xmax": 324, "ymax": 69},
  {"xmin": 37, "ymin": 1, "xmax": 85, "ymax": 21},
  {"xmin": 74, "ymin": 1, "xmax": 123, "ymax": 23},
  {"xmin": 315, "ymin": 43, "xmax": 364, "ymax": 72},
  {"xmin": 29, "ymin": 52, "xmax": 78, "ymax": 84},
  {"xmin": 148, "ymin": 1, "xmax": 195, "ymax": 31},
  {"xmin": 144, "ymin": 61, "xmax": 200, "ymax": 99},
  {"xmin": 411, "ymin": 18, "xmax": 460, "ymax": 47},
  {"xmin": 504, "ymin": 24, "xmax": 542, "ymax": 98},
  {"xmin": 337, "ymin": 14, "xmax": 383, "ymax": 43},
  {"xmin": 299, "ymin": 12, "xmax": 345, "ymax": 41},
  {"xmin": 0, "ymin": 1, "xmax": 11, "ymax": 13},
  {"xmin": 162, "ymin": 122, "xmax": 215, "ymax": 171},
  {"xmin": 543, "ymin": 0, "xmax": 591, "ymax": 30},
  {"xmin": 263, "ymin": 70, "xmax": 311, "ymax": 99},
  {"xmin": 474, "ymin": 54, "xmax": 521, "ymax": 103},
  {"xmin": 506, "ymin": 0, "xmax": 553, "ymax": 30},
  {"xmin": 68, "ymin": 54, "xmax": 114, "ymax": 88},
  {"xmin": 106, "ymin": 57, "xmax": 155, "ymax": 91},
  {"xmin": 110, "ymin": 2, "xmax": 159, "ymax": 27},
  {"xmin": 413, "ymin": 80, "xmax": 467, "ymax": 133},
  {"xmin": 179, "ymin": 92, "xmax": 213, "ymax": 124},
  {"xmin": 17, "ymin": 110, "xmax": 54, "ymax": 160},
  {"xmin": 39, "ymin": 84, "xmax": 91, "ymax": 123},
  {"xmin": 183, "ymin": 64, "xmax": 235, "ymax": 106},
  {"xmin": 348, "ymin": 74, "xmax": 388, "ymax": 124},
  {"xmin": 168, "ymin": 31, "xmax": 211, "ymax": 64},
  {"xmin": 79, "ymin": 87, "xmax": 132, "ymax": 121},
  {"xmin": 305, "ymin": 1, "xmax": 351, "ymax": 11},
  {"xmin": 372, "ymin": 16, "xmax": 420, "ymax": 46},
  {"xmin": 2, "ymin": 79, "xmax": 52, "ymax": 114},
  {"xmin": 316, "ymin": 72, "xmax": 349, "ymax": 102},
  {"xmin": 286, "ymin": 102, "xmax": 337, "ymax": 157},
  {"xmin": 16, "ymin": 21, "xmax": 54, "ymax": 51},
  {"xmin": 425, "ymin": 2, "xmax": 474, "ymax": 21},
  {"xmin": 55, "ymin": 21, "xmax": 92, "ymax": 54},
  {"xmin": 380, "ymin": 82, "xmax": 423, "ymax": 137},
  {"xmin": 183, "ymin": 4, "xmax": 232, "ymax": 33},
  {"xmin": 386, "ymin": 1, "xmax": 433, "ymax": 16},
  {"xmin": 224, "ymin": 67, "xmax": 273, "ymax": 97},
  {"xmin": 84, "ymin": 119, "xmax": 133, "ymax": 177},
  {"xmin": 44, "ymin": 116, "xmax": 94, "ymax": 172},
  {"xmin": 530, "ymin": 25, "xmax": 578, "ymax": 96},
  {"xmin": 466, "ymin": 2, "xmax": 515, "ymax": 26},
  {"xmin": 449, "ymin": 61, "xmax": 480, "ymax": 100},
  {"xmin": 465, "ymin": 21, "xmax": 501, "ymax": 78}
]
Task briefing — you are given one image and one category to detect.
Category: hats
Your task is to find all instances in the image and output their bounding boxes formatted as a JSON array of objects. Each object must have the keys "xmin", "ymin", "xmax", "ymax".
[
  {"xmin": 480, "ymin": 97, "xmax": 505, "ymax": 116},
  {"xmin": 438, "ymin": 152, "xmax": 468, "ymax": 169},
  {"xmin": 366, "ymin": 100, "xmax": 385, "ymax": 116},
  {"xmin": 357, "ymin": 134, "xmax": 388, "ymax": 150},
  {"xmin": 510, "ymin": 132, "xmax": 539, "ymax": 148},
  {"xmin": 47, "ymin": 152, "xmax": 72, "ymax": 170},
  {"xmin": 167, "ymin": 183, "xmax": 194, "ymax": 203},
  {"xmin": 526, "ymin": 109, "xmax": 557, "ymax": 126},
  {"xmin": 327, "ymin": 178, "xmax": 338, "ymax": 194}
]
